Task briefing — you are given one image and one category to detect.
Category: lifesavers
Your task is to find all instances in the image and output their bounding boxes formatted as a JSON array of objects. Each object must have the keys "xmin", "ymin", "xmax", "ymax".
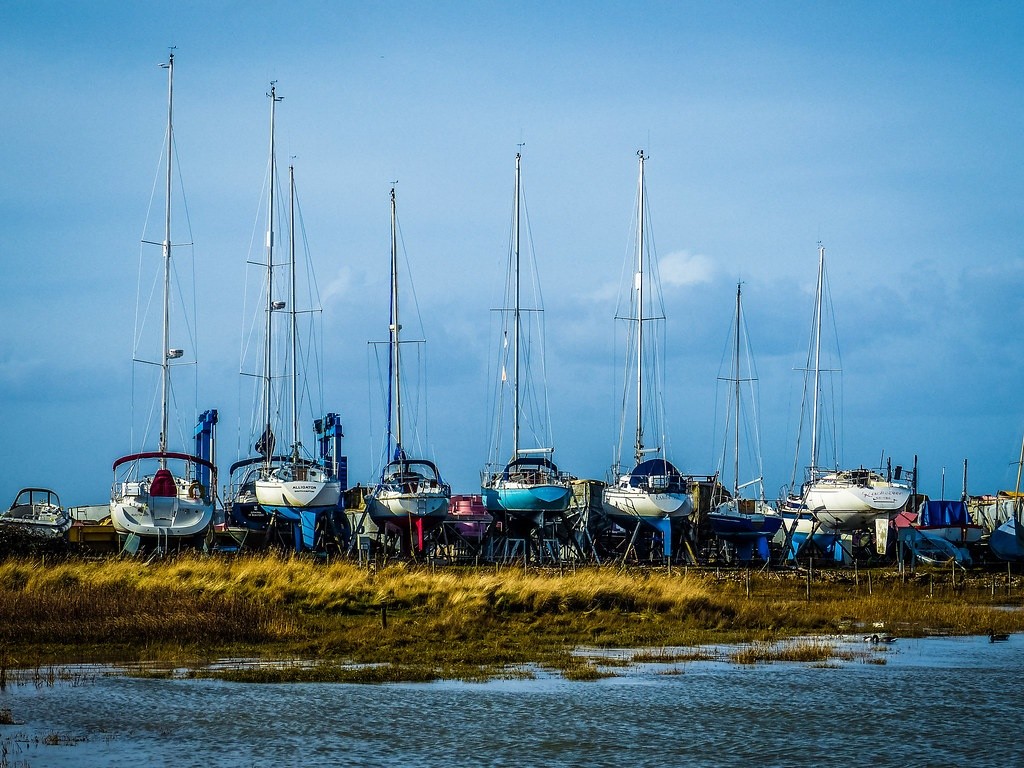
[{"xmin": 188, "ymin": 482, "xmax": 205, "ymax": 499}]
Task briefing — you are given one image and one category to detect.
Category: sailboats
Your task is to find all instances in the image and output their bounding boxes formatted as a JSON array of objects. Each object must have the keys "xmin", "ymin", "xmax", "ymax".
[
  {"xmin": 255, "ymin": 87, "xmax": 342, "ymax": 547},
  {"xmin": 781, "ymin": 247, "xmax": 839, "ymax": 549},
  {"xmin": 363, "ymin": 187, "xmax": 451, "ymax": 551},
  {"xmin": 708, "ymin": 284, "xmax": 784, "ymax": 561},
  {"xmin": 109, "ymin": 53, "xmax": 217, "ymax": 541},
  {"xmin": 481, "ymin": 152, "xmax": 573, "ymax": 529},
  {"xmin": 601, "ymin": 149, "xmax": 692, "ymax": 557}
]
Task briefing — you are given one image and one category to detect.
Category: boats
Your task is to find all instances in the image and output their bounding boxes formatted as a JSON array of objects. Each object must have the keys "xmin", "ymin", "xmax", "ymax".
[
  {"xmin": 908, "ymin": 499, "xmax": 983, "ymax": 544},
  {"xmin": 0, "ymin": 487, "xmax": 72, "ymax": 538},
  {"xmin": 803, "ymin": 467, "xmax": 910, "ymax": 555},
  {"xmin": 988, "ymin": 517, "xmax": 1024, "ymax": 562}
]
